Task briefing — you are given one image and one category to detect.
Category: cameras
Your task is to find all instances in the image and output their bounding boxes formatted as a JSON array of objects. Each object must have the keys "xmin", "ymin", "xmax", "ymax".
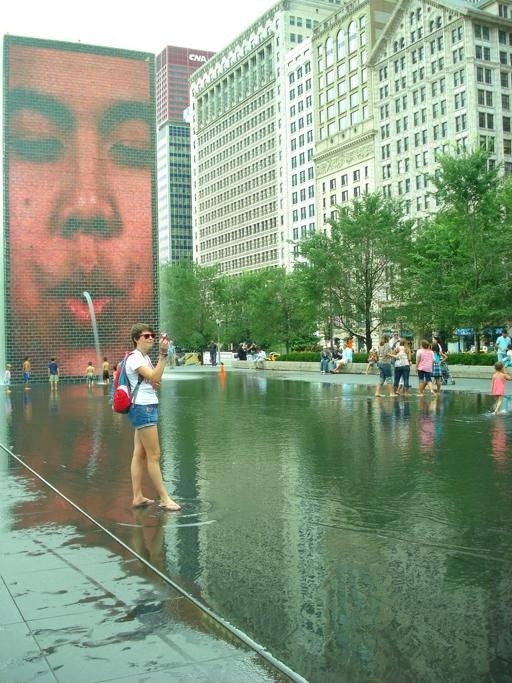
[{"xmin": 160, "ymin": 332, "xmax": 169, "ymax": 343}]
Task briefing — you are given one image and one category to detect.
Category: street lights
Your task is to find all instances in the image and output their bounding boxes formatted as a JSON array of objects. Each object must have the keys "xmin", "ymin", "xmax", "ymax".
[{"xmin": 216, "ymin": 318, "xmax": 225, "ymax": 364}]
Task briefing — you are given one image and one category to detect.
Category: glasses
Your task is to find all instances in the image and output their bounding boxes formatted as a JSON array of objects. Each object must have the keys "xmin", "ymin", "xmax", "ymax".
[{"xmin": 137, "ymin": 333, "xmax": 156, "ymax": 340}]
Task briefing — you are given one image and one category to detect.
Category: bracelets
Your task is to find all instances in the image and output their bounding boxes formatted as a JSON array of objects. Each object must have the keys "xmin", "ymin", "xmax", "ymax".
[{"xmin": 159, "ymin": 351, "xmax": 168, "ymax": 356}]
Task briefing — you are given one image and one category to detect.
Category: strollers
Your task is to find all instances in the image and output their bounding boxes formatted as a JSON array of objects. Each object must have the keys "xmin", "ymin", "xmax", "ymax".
[{"xmin": 440, "ymin": 359, "xmax": 455, "ymax": 386}]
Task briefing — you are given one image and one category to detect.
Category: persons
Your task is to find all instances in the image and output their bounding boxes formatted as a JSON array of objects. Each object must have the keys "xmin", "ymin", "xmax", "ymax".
[
  {"xmin": 49, "ymin": 392, "xmax": 60, "ymax": 416},
  {"xmin": 48, "ymin": 357, "xmax": 60, "ymax": 392},
  {"xmin": 22, "ymin": 356, "xmax": 32, "ymax": 390},
  {"xmin": 4, "ymin": 363, "xmax": 12, "ymax": 394},
  {"xmin": 490, "ymin": 361, "xmax": 512, "ymax": 415},
  {"xmin": 23, "ymin": 390, "xmax": 32, "ymax": 423},
  {"xmin": 238, "ymin": 341, "xmax": 267, "ymax": 370},
  {"xmin": 86, "ymin": 362, "xmax": 95, "ymax": 388},
  {"xmin": 208, "ymin": 340, "xmax": 218, "ymax": 368},
  {"xmin": 9, "ymin": 41, "xmax": 155, "ymax": 375},
  {"xmin": 122, "ymin": 322, "xmax": 182, "ymax": 512},
  {"xmin": 373, "ymin": 398, "xmax": 446, "ymax": 461},
  {"xmin": 365, "ymin": 331, "xmax": 449, "ymax": 396},
  {"xmin": 102, "ymin": 356, "xmax": 110, "ymax": 385},
  {"xmin": 504, "ymin": 344, "xmax": 512, "ymax": 368},
  {"xmin": 320, "ymin": 340, "xmax": 352, "ymax": 374},
  {"xmin": 167, "ymin": 340, "xmax": 176, "ymax": 369},
  {"xmin": 490, "ymin": 416, "xmax": 507, "ymax": 465},
  {"xmin": 129, "ymin": 513, "xmax": 170, "ymax": 579},
  {"xmin": 495, "ymin": 329, "xmax": 511, "ymax": 362}
]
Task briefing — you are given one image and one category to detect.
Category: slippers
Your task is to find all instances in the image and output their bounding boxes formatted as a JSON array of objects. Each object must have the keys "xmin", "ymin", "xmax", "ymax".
[
  {"xmin": 131, "ymin": 497, "xmax": 155, "ymax": 507},
  {"xmin": 158, "ymin": 503, "xmax": 181, "ymax": 512}
]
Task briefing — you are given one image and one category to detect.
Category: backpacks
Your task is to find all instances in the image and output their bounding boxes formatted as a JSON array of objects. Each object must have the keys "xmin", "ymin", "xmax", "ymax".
[{"xmin": 113, "ymin": 350, "xmax": 145, "ymax": 414}]
